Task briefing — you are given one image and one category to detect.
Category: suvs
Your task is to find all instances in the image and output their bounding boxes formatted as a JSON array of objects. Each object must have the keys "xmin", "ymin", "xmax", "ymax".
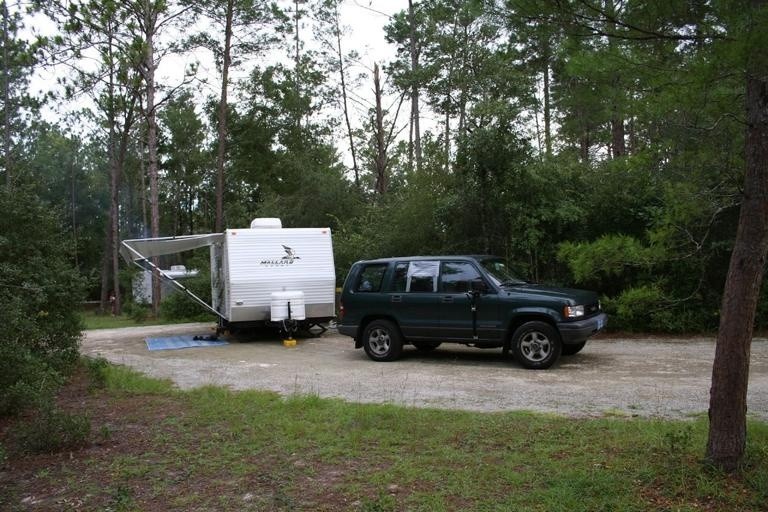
[{"xmin": 338, "ymin": 254, "xmax": 607, "ymax": 369}]
